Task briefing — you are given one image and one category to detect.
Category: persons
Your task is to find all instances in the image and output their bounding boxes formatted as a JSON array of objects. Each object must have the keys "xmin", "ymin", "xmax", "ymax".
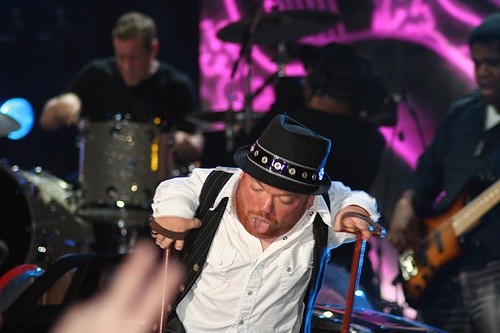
[
  {"xmin": 231, "ymin": 42, "xmax": 380, "ymax": 193},
  {"xmin": 149, "ymin": 116, "xmax": 382, "ymax": 333},
  {"xmin": 55, "ymin": 239, "xmax": 184, "ymax": 333},
  {"xmin": 41, "ymin": 12, "xmax": 205, "ymax": 180},
  {"xmin": 386, "ymin": 15, "xmax": 500, "ymax": 333}
]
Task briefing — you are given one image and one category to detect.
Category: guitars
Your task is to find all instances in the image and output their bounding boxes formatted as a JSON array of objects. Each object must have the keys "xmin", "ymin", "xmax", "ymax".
[{"xmin": 400, "ymin": 179, "xmax": 500, "ymax": 303}]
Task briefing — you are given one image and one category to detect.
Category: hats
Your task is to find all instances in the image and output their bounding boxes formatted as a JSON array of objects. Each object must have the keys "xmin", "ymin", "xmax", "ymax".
[
  {"xmin": 234, "ymin": 113, "xmax": 332, "ymax": 196},
  {"xmin": 300, "ymin": 41, "xmax": 363, "ymax": 87}
]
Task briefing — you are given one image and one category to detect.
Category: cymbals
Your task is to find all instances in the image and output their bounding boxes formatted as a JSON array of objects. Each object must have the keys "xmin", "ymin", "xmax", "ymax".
[{"xmin": 217, "ymin": 9, "xmax": 341, "ymax": 44}]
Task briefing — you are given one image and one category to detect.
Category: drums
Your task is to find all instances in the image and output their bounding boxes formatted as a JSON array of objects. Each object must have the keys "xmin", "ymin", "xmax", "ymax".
[
  {"xmin": 77, "ymin": 113, "xmax": 180, "ymax": 223},
  {"xmin": 2, "ymin": 164, "xmax": 93, "ymax": 267}
]
